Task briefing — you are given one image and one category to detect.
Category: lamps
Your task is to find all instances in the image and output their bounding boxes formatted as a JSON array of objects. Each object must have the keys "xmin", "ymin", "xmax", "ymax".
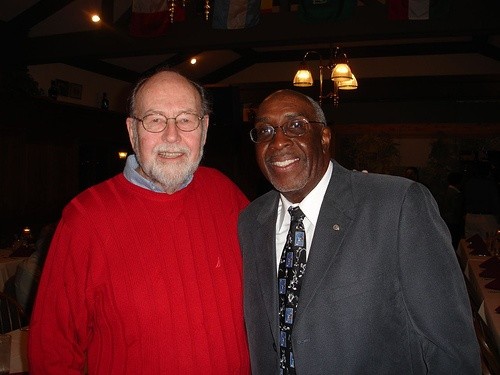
[{"xmin": 292, "ymin": 46, "xmax": 360, "ymax": 107}]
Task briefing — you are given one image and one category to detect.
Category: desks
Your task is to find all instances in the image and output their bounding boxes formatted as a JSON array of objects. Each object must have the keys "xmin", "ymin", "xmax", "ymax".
[
  {"xmin": 0, "ymin": 247, "xmax": 31, "ymax": 293},
  {"xmin": 456, "ymin": 239, "xmax": 500, "ymax": 355},
  {"xmin": 0, "ymin": 323, "xmax": 30, "ymax": 375}
]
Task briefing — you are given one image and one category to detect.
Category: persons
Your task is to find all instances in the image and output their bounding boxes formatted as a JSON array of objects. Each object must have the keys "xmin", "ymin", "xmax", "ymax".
[
  {"xmin": 239, "ymin": 89, "xmax": 482, "ymax": 375},
  {"xmin": 12, "ymin": 197, "xmax": 70, "ymax": 329},
  {"xmin": 26, "ymin": 70, "xmax": 252, "ymax": 375},
  {"xmin": 405, "ymin": 147, "xmax": 500, "ymax": 249}
]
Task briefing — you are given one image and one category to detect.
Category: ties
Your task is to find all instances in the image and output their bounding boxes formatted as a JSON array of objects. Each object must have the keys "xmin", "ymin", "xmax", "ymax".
[{"xmin": 277, "ymin": 206, "xmax": 306, "ymax": 375}]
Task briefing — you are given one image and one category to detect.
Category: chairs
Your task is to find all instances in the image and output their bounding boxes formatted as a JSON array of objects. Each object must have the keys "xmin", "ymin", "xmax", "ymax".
[{"xmin": 0, "ymin": 293, "xmax": 29, "ymax": 333}]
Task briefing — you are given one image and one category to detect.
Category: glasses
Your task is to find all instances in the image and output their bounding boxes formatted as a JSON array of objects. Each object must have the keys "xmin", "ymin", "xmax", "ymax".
[
  {"xmin": 250, "ymin": 117, "xmax": 326, "ymax": 143},
  {"xmin": 133, "ymin": 114, "xmax": 205, "ymax": 133}
]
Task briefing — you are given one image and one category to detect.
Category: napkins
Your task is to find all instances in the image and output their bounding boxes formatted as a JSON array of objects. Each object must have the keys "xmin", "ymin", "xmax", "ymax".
[{"xmin": 467, "ymin": 234, "xmax": 500, "ymax": 314}]
